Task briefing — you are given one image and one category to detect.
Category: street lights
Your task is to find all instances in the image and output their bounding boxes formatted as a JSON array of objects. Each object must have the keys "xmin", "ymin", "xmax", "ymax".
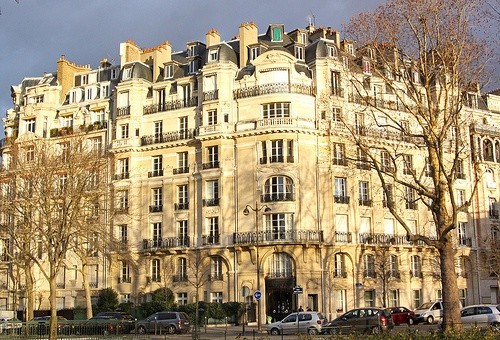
[{"xmin": 243, "ymin": 201, "xmax": 275, "ymax": 331}]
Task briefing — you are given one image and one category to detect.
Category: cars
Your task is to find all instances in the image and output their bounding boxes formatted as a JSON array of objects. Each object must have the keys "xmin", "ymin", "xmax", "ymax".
[
  {"xmin": 414, "ymin": 301, "xmax": 443, "ymax": 325},
  {"xmin": 385, "ymin": 307, "xmax": 416, "ymax": 325},
  {"xmin": 23, "ymin": 311, "xmax": 136, "ymax": 337},
  {"xmin": 268, "ymin": 312, "xmax": 325, "ymax": 336},
  {"xmin": 460, "ymin": 303, "xmax": 500, "ymax": 332},
  {"xmin": 324, "ymin": 307, "xmax": 395, "ymax": 335},
  {"xmin": 135, "ymin": 311, "xmax": 190, "ymax": 334}
]
